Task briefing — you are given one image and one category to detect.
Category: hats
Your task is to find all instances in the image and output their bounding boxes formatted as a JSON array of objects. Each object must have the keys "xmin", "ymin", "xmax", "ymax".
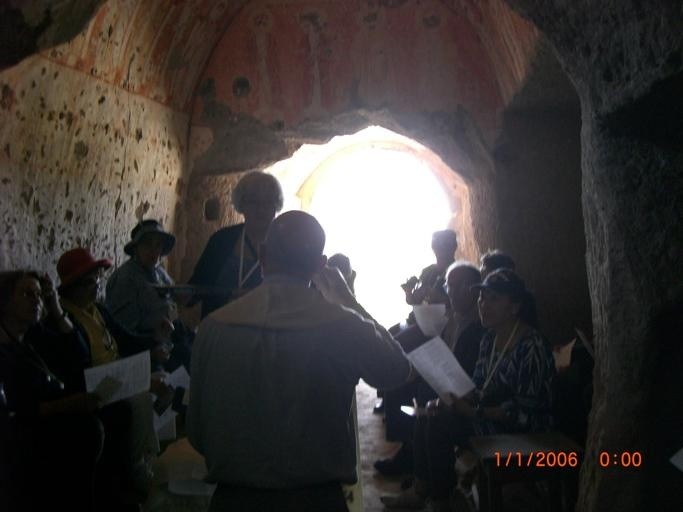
[
  {"xmin": 124, "ymin": 220, "xmax": 175, "ymax": 255},
  {"xmin": 472, "ymin": 269, "xmax": 524, "ymax": 296},
  {"xmin": 57, "ymin": 249, "xmax": 111, "ymax": 282}
]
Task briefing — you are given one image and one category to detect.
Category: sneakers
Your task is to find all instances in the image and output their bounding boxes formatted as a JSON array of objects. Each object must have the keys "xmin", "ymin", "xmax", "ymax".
[{"xmin": 374, "ymin": 459, "xmax": 445, "ymax": 512}]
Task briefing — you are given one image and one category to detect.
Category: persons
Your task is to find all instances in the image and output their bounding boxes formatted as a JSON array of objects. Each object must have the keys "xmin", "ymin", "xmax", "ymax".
[
  {"xmin": 373, "ymin": 230, "xmax": 559, "ymax": 511},
  {"xmin": 0, "ymin": 170, "xmax": 412, "ymax": 509}
]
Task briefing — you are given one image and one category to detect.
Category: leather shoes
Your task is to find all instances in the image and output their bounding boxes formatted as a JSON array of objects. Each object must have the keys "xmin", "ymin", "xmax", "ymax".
[{"xmin": 374, "ymin": 397, "xmax": 384, "ymax": 411}]
